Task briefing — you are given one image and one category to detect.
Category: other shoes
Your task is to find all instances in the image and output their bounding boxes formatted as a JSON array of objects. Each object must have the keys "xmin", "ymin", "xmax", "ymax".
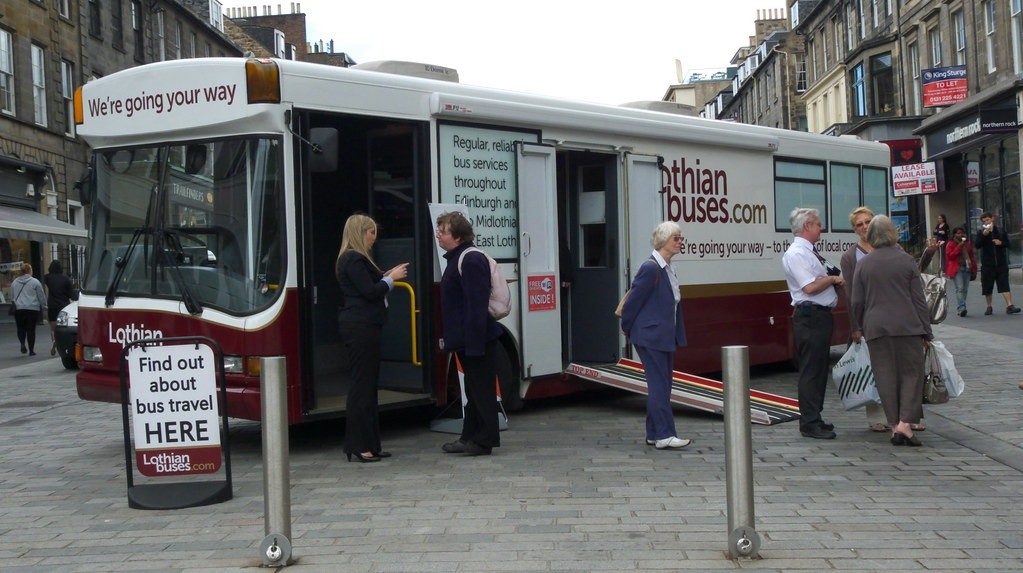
[
  {"xmin": 29, "ymin": 352, "xmax": 35, "ymax": 355},
  {"xmin": 21, "ymin": 345, "xmax": 27, "ymax": 353},
  {"xmin": 443, "ymin": 439, "xmax": 492, "ymax": 455},
  {"xmin": 51, "ymin": 340, "xmax": 56, "ymax": 355},
  {"xmin": 985, "ymin": 308, "xmax": 992, "ymax": 315},
  {"xmin": 872, "ymin": 422, "xmax": 887, "ymax": 432},
  {"xmin": 910, "ymin": 423, "xmax": 925, "ymax": 431},
  {"xmin": 1007, "ymin": 306, "xmax": 1021, "ymax": 315},
  {"xmin": 959, "ymin": 310, "xmax": 967, "ymax": 317},
  {"xmin": 645, "ymin": 435, "xmax": 691, "ymax": 449}
]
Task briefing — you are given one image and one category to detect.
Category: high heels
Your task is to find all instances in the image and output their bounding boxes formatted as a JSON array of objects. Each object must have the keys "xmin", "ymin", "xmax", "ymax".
[
  {"xmin": 376, "ymin": 451, "xmax": 391, "ymax": 458},
  {"xmin": 346, "ymin": 449, "xmax": 381, "ymax": 462}
]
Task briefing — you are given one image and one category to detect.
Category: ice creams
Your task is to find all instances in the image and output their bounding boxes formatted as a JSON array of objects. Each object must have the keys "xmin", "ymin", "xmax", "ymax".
[
  {"xmin": 982, "ymin": 224, "xmax": 990, "ymax": 229},
  {"xmin": 962, "ymin": 238, "xmax": 966, "ymax": 241}
]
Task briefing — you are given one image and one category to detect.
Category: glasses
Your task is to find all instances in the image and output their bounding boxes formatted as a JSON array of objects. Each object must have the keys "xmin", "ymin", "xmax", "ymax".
[
  {"xmin": 670, "ymin": 236, "xmax": 685, "ymax": 242},
  {"xmin": 855, "ymin": 219, "xmax": 872, "ymax": 228}
]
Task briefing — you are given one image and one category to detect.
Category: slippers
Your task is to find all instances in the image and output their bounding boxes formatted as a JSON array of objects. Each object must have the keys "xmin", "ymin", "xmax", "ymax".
[{"xmin": 891, "ymin": 431, "xmax": 923, "ymax": 446}]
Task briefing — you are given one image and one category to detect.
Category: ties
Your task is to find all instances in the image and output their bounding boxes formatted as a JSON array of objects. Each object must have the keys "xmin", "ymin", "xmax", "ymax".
[{"xmin": 813, "ymin": 246, "xmax": 838, "ymax": 293}]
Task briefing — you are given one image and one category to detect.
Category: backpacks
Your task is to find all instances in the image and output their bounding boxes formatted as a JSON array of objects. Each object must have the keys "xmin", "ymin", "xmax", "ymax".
[{"xmin": 458, "ymin": 248, "xmax": 512, "ymax": 320}]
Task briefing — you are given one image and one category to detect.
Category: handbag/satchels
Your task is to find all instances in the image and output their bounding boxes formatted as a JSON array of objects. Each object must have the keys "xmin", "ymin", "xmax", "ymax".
[
  {"xmin": 922, "ymin": 340, "xmax": 965, "ymax": 404},
  {"xmin": 8, "ymin": 302, "xmax": 16, "ymax": 315},
  {"xmin": 916, "ymin": 244, "xmax": 949, "ymax": 324},
  {"xmin": 614, "ymin": 288, "xmax": 632, "ymax": 319},
  {"xmin": 832, "ymin": 336, "xmax": 881, "ymax": 412}
]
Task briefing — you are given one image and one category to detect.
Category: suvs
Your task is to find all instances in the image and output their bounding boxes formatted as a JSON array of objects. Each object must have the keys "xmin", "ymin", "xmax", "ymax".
[{"xmin": 56, "ymin": 244, "xmax": 214, "ymax": 368}]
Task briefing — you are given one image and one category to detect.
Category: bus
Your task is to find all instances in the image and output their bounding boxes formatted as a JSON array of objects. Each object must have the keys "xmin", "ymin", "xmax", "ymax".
[{"xmin": 74, "ymin": 52, "xmax": 891, "ymax": 427}]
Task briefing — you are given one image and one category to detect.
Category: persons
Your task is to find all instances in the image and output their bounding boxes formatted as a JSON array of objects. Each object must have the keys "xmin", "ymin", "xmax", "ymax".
[
  {"xmin": 974, "ymin": 212, "xmax": 1022, "ymax": 315},
  {"xmin": 932, "ymin": 214, "xmax": 950, "ymax": 273},
  {"xmin": 850, "ymin": 212, "xmax": 935, "ymax": 447},
  {"xmin": 946, "ymin": 227, "xmax": 979, "ymax": 317},
  {"xmin": 841, "ymin": 207, "xmax": 929, "ymax": 432},
  {"xmin": 434, "ymin": 207, "xmax": 502, "ymax": 456},
  {"xmin": 9, "ymin": 263, "xmax": 48, "ymax": 358},
  {"xmin": 780, "ymin": 206, "xmax": 847, "ymax": 439},
  {"xmin": 620, "ymin": 220, "xmax": 691, "ymax": 450},
  {"xmin": 45, "ymin": 259, "xmax": 78, "ymax": 357},
  {"xmin": 333, "ymin": 215, "xmax": 409, "ymax": 462}
]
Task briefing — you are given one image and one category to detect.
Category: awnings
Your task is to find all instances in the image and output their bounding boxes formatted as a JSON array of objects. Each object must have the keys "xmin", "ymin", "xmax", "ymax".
[{"xmin": 0, "ymin": 205, "xmax": 89, "ymax": 247}]
